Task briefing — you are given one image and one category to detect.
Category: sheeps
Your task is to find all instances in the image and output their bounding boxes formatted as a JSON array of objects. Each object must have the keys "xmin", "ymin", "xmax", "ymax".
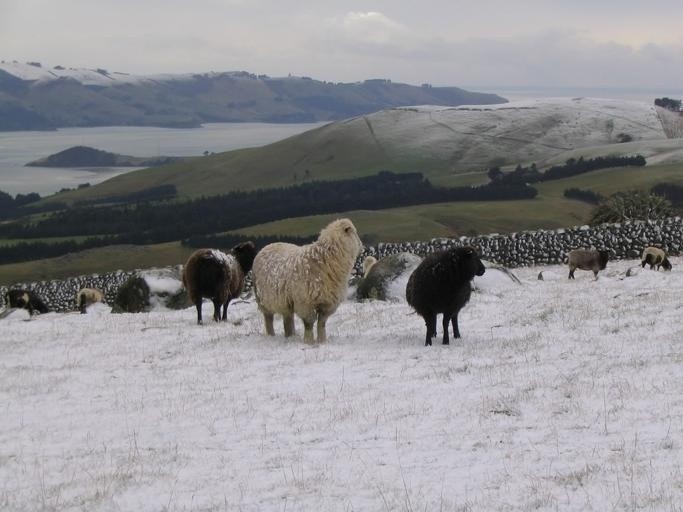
[
  {"xmin": 4, "ymin": 290, "xmax": 49, "ymax": 315},
  {"xmin": 567, "ymin": 249, "xmax": 609, "ymax": 281},
  {"xmin": 250, "ymin": 218, "xmax": 365, "ymax": 346},
  {"xmin": 404, "ymin": 244, "xmax": 486, "ymax": 347},
  {"xmin": 78, "ymin": 286, "xmax": 102, "ymax": 314},
  {"xmin": 184, "ymin": 241, "xmax": 257, "ymax": 324},
  {"xmin": 640, "ymin": 247, "xmax": 672, "ymax": 272},
  {"xmin": 361, "ymin": 256, "xmax": 376, "ymax": 273}
]
